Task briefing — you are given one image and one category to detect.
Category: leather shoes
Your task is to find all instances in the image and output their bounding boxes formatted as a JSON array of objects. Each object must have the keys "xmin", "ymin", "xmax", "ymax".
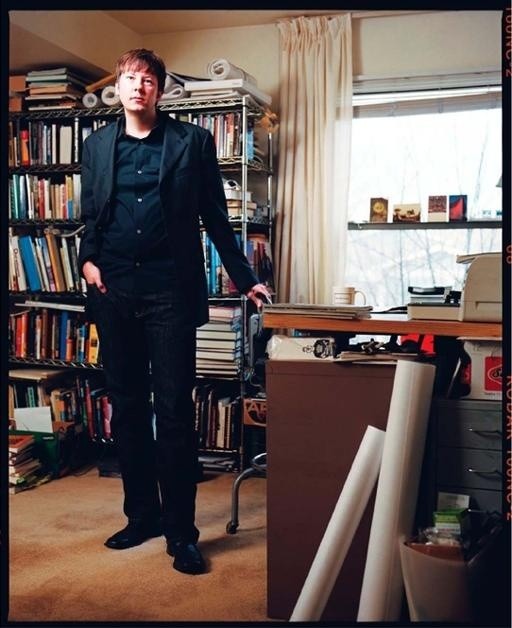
[
  {"xmin": 102, "ymin": 516, "xmax": 166, "ymax": 550},
  {"xmin": 163, "ymin": 533, "xmax": 206, "ymax": 575}
]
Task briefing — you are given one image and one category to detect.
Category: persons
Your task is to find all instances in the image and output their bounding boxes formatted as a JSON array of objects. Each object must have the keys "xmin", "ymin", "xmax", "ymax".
[{"xmin": 78, "ymin": 48, "xmax": 274, "ymax": 575}]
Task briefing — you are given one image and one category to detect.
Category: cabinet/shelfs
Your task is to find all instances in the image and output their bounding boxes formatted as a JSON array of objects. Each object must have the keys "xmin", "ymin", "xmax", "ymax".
[
  {"xmin": 8, "ymin": 98, "xmax": 273, "ymax": 479},
  {"xmin": 437, "ymin": 399, "xmax": 503, "ymax": 533}
]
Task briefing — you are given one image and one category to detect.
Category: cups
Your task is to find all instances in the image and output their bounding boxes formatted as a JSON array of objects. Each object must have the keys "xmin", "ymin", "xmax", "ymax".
[{"xmin": 332, "ymin": 287, "xmax": 366, "ymax": 307}]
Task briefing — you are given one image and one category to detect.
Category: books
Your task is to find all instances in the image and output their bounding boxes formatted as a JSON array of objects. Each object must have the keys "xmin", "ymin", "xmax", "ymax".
[
  {"xmin": 260, "ymin": 301, "xmax": 373, "ymax": 320},
  {"xmin": 339, "ymin": 351, "xmax": 417, "ymax": 363},
  {"xmin": 7, "ymin": 65, "xmax": 272, "ymax": 494}
]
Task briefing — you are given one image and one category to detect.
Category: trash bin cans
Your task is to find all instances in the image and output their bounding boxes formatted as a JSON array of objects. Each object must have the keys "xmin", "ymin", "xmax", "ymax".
[{"xmin": 398, "ymin": 526, "xmax": 503, "ymax": 622}]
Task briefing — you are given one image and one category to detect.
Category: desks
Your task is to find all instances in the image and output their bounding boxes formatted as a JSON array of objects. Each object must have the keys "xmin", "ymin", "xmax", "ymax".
[{"xmin": 261, "ymin": 311, "xmax": 503, "ymax": 619}]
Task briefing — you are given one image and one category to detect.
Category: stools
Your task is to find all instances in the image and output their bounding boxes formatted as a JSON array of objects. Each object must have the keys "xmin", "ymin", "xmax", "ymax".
[{"xmin": 226, "ymin": 452, "xmax": 267, "ymax": 535}]
